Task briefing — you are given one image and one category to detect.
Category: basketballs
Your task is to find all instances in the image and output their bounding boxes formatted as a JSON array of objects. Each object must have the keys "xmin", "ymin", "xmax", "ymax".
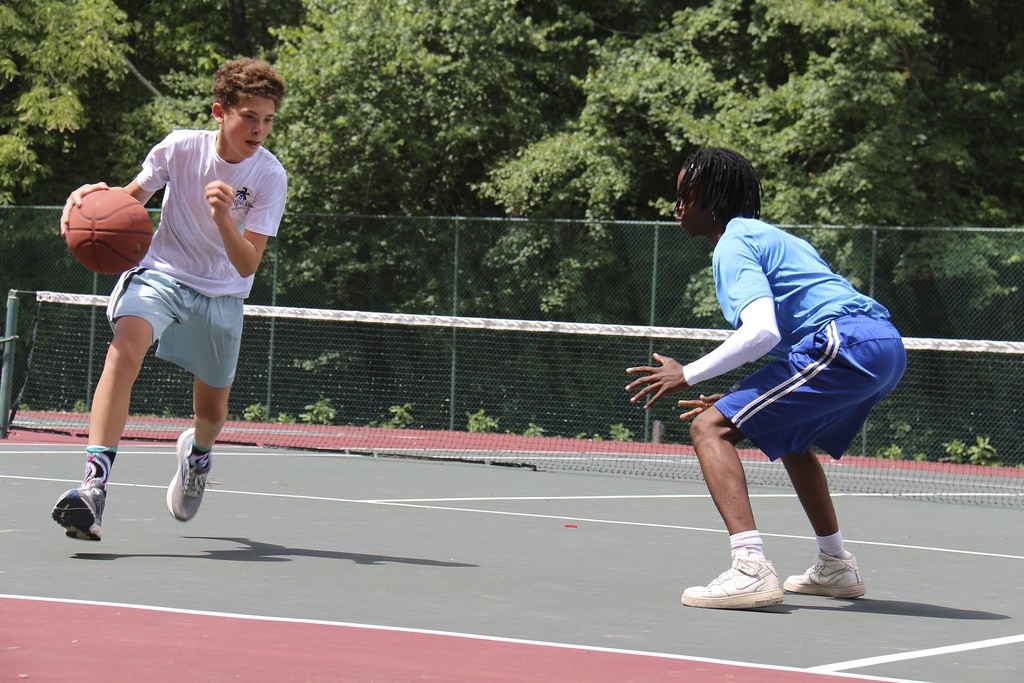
[{"xmin": 64, "ymin": 189, "xmax": 152, "ymax": 276}]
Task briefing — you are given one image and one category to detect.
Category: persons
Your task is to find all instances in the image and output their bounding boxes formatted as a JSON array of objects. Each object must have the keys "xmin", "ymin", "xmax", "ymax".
[
  {"xmin": 622, "ymin": 148, "xmax": 908, "ymax": 611},
  {"xmin": 53, "ymin": 60, "xmax": 289, "ymax": 542}
]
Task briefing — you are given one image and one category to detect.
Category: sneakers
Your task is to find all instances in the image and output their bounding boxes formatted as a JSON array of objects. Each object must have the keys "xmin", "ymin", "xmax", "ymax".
[
  {"xmin": 784, "ymin": 550, "xmax": 865, "ymax": 598},
  {"xmin": 681, "ymin": 558, "xmax": 782, "ymax": 609},
  {"xmin": 166, "ymin": 429, "xmax": 212, "ymax": 520},
  {"xmin": 50, "ymin": 479, "xmax": 107, "ymax": 541}
]
